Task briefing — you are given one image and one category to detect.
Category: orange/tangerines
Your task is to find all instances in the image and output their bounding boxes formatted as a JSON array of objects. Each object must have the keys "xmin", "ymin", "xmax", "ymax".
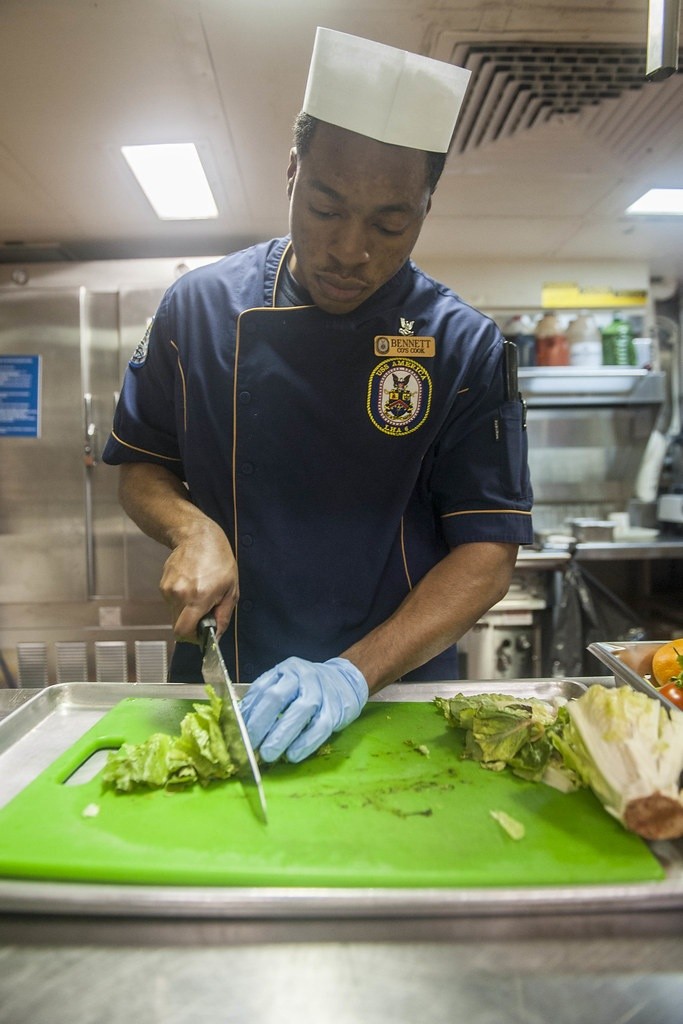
[{"xmin": 652, "ymin": 638, "xmax": 683, "ymax": 685}]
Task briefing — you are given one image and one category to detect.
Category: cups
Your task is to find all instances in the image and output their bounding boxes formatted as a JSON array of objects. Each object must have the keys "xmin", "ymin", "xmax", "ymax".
[{"xmin": 632, "ymin": 338, "xmax": 651, "ymax": 367}]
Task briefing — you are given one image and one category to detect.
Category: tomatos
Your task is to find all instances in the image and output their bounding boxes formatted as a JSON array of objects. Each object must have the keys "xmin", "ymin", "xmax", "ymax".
[{"xmin": 659, "ymin": 683, "xmax": 683, "ymax": 712}]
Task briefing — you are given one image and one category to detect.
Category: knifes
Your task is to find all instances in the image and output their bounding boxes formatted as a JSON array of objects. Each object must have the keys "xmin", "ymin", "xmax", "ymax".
[{"xmin": 197, "ymin": 612, "xmax": 271, "ymax": 824}]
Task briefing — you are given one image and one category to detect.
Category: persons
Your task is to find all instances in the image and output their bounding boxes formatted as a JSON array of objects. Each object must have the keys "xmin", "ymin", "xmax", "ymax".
[{"xmin": 107, "ymin": 99, "xmax": 532, "ymax": 765}]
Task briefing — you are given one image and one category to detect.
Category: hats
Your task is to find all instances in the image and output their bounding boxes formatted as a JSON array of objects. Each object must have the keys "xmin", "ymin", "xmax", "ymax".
[{"xmin": 303, "ymin": 27, "xmax": 472, "ymax": 154}]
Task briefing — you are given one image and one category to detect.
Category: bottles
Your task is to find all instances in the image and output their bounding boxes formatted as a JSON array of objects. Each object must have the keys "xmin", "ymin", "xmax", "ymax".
[
  {"xmin": 565, "ymin": 311, "xmax": 602, "ymax": 365},
  {"xmin": 536, "ymin": 312, "xmax": 569, "ymax": 366},
  {"xmin": 603, "ymin": 314, "xmax": 635, "ymax": 365},
  {"xmin": 502, "ymin": 316, "xmax": 536, "ymax": 366}
]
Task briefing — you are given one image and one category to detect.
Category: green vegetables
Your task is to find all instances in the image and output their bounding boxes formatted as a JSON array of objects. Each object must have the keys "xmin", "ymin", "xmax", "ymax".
[
  {"xmin": 101, "ymin": 681, "xmax": 330, "ymax": 791},
  {"xmin": 438, "ymin": 686, "xmax": 683, "ymax": 841}
]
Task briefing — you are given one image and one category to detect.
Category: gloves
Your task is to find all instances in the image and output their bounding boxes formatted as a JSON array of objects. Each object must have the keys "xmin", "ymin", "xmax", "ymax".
[{"xmin": 238, "ymin": 657, "xmax": 368, "ymax": 763}]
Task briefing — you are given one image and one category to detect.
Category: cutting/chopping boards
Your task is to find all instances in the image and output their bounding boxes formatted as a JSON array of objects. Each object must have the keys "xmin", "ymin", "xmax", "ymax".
[{"xmin": 0, "ymin": 696, "xmax": 662, "ymax": 887}]
